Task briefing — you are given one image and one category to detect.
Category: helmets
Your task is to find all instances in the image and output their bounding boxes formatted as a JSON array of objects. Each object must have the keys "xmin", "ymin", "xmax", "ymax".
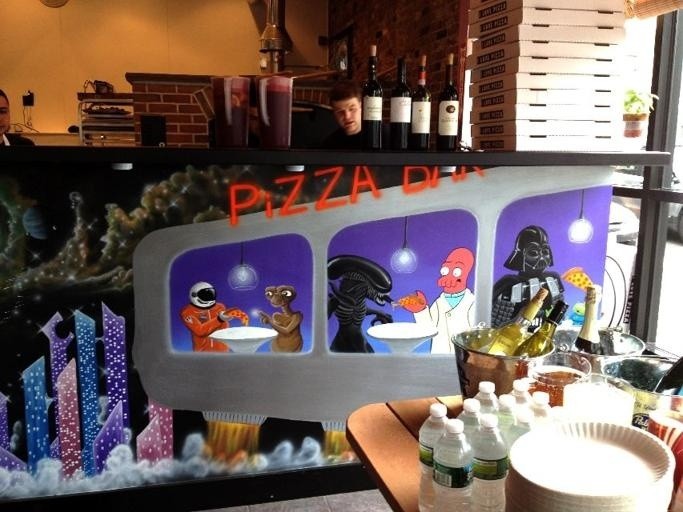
[
  {"xmin": 504, "ymin": 226, "xmax": 553, "ymax": 269},
  {"xmin": 190, "ymin": 282, "xmax": 217, "ymax": 310}
]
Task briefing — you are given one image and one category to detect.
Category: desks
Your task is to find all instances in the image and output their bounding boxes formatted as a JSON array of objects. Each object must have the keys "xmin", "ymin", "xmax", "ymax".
[{"xmin": 346, "ymin": 390, "xmax": 516, "ymax": 512}]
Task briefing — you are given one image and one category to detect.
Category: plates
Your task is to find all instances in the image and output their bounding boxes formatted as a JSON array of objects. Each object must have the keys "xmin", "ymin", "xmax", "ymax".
[{"xmin": 505, "ymin": 419, "xmax": 676, "ymax": 512}]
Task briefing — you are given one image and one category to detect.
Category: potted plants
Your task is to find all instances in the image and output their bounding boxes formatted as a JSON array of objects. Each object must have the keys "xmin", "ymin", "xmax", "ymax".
[{"xmin": 620, "ymin": 89, "xmax": 666, "ymax": 143}]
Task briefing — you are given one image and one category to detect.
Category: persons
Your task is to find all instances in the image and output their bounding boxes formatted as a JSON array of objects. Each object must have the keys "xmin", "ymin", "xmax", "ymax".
[
  {"xmin": 1, "ymin": 90, "xmax": 35, "ymax": 146},
  {"xmin": 323, "ymin": 88, "xmax": 390, "ymax": 152}
]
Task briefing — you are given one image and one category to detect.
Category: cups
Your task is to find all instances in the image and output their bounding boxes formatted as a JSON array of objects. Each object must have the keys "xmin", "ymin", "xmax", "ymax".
[
  {"xmin": 211, "ymin": 75, "xmax": 249, "ymax": 147},
  {"xmin": 256, "ymin": 77, "xmax": 290, "ymax": 148}
]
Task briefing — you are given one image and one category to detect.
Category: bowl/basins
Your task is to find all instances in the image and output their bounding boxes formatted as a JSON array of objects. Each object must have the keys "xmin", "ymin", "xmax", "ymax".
[
  {"xmin": 532, "ymin": 355, "xmax": 592, "ymax": 402},
  {"xmin": 368, "ymin": 320, "xmax": 440, "ymax": 352},
  {"xmin": 207, "ymin": 325, "xmax": 281, "ymax": 354}
]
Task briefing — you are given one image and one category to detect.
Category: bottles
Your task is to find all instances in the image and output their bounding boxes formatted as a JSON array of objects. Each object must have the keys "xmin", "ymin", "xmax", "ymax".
[
  {"xmin": 411, "ymin": 54, "xmax": 430, "ymax": 147},
  {"xmin": 578, "ymin": 285, "xmax": 600, "ymax": 356},
  {"xmin": 488, "ymin": 287, "xmax": 547, "ymax": 360},
  {"xmin": 437, "ymin": 54, "xmax": 457, "ymax": 148},
  {"xmin": 363, "ymin": 46, "xmax": 383, "ymax": 149},
  {"xmin": 392, "ymin": 56, "xmax": 408, "ymax": 149},
  {"xmin": 417, "ymin": 378, "xmax": 551, "ymax": 512},
  {"xmin": 516, "ymin": 298, "xmax": 570, "ymax": 357}
]
